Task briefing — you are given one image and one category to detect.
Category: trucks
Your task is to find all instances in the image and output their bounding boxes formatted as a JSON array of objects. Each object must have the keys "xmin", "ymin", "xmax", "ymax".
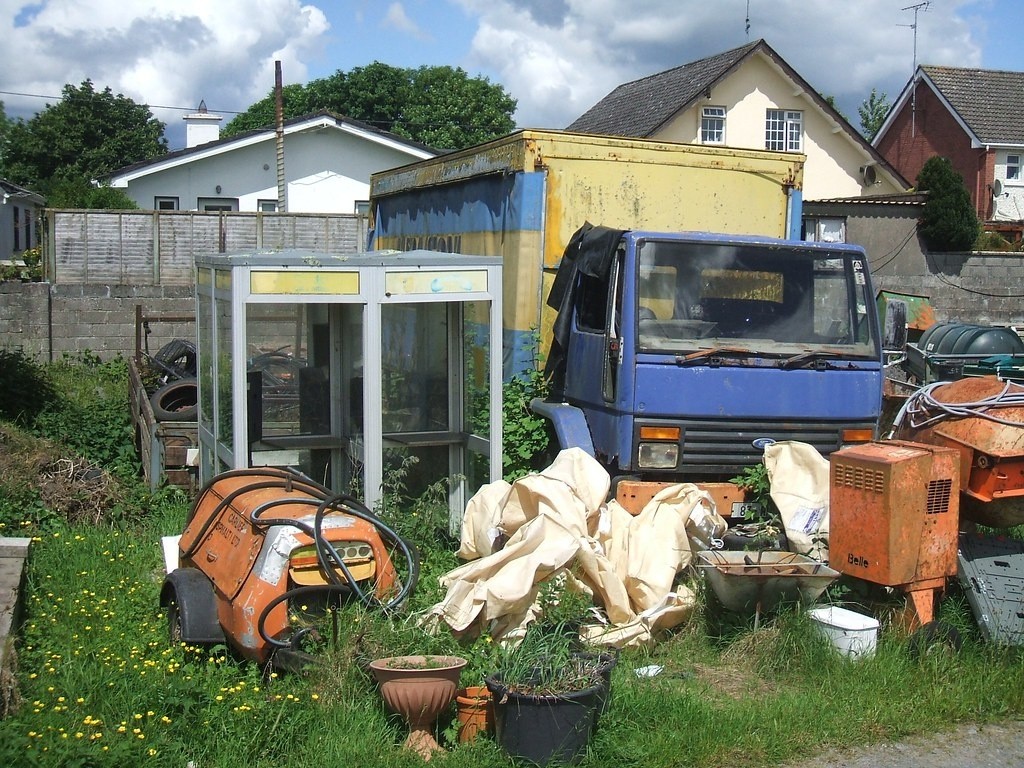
[{"xmin": 365, "ymin": 125, "xmax": 911, "ymax": 525}]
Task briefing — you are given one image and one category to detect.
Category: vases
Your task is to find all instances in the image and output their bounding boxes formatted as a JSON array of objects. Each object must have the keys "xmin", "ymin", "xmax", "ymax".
[{"xmin": 454, "ymin": 685, "xmax": 494, "ymax": 745}]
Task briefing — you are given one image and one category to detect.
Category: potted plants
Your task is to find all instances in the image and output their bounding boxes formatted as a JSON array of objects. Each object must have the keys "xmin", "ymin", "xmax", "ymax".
[
  {"xmin": 485, "ymin": 618, "xmax": 604, "ymax": 768},
  {"xmin": 525, "ymin": 557, "xmax": 592, "ymax": 642},
  {"xmin": 535, "ymin": 575, "xmax": 618, "ymax": 735},
  {"xmin": 369, "ymin": 654, "xmax": 468, "ymax": 762}
]
